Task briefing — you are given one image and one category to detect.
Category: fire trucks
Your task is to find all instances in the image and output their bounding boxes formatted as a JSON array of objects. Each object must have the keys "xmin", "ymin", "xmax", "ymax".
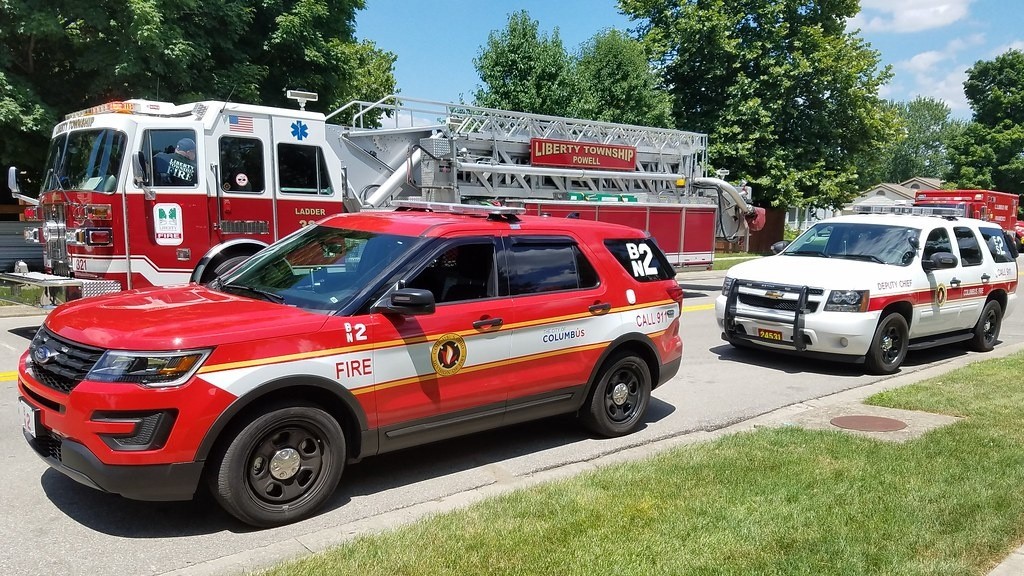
[{"xmin": 7, "ymin": 89, "xmax": 760, "ymax": 320}]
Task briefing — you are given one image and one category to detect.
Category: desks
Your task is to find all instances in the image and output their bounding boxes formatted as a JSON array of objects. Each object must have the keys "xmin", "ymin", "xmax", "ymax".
[{"xmin": 0, "ymin": 271, "xmax": 85, "ymax": 310}]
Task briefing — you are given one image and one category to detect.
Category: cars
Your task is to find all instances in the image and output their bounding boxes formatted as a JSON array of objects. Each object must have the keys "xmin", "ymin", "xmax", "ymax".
[{"xmin": 1014, "ymin": 219, "xmax": 1024, "ymax": 254}]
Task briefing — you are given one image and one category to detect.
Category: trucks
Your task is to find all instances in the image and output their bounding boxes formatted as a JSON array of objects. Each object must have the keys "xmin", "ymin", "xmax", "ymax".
[{"xmin": 911, "ymin": 188, "xmax": 1020, "ymax": 248}]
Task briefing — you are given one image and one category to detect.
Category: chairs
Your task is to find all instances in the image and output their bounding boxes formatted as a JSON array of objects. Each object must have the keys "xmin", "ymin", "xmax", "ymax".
[{"xmin": 445, "ymin": 255, "xmax": 489, "ymax": 304}]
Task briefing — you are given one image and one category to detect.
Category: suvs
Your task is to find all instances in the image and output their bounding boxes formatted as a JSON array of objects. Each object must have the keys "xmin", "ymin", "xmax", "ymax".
[
  {"xmin": 715, "ymin": 203, "xmax": 1018, "ymax": 375},
  {"xmin": 12, "ymin": 203, "xmax": 686, "ymax": 528}
]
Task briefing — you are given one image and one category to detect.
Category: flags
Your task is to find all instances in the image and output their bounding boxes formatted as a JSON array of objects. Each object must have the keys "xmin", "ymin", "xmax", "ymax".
[{"xmin": 228, "ymin": 114, "xmax": 253, "ymax": 133}]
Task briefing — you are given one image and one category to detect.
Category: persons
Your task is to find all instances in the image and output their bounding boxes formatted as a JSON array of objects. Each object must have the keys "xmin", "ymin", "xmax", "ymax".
[{"xmin": 152, "ymin": 137, "xmax": 196, "ymax": 187}]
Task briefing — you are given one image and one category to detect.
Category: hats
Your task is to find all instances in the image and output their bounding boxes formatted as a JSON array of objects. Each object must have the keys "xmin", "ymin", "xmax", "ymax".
[{"xmin": 176, "ymin": 138, "xmax": 196, "ymax": 151}]
